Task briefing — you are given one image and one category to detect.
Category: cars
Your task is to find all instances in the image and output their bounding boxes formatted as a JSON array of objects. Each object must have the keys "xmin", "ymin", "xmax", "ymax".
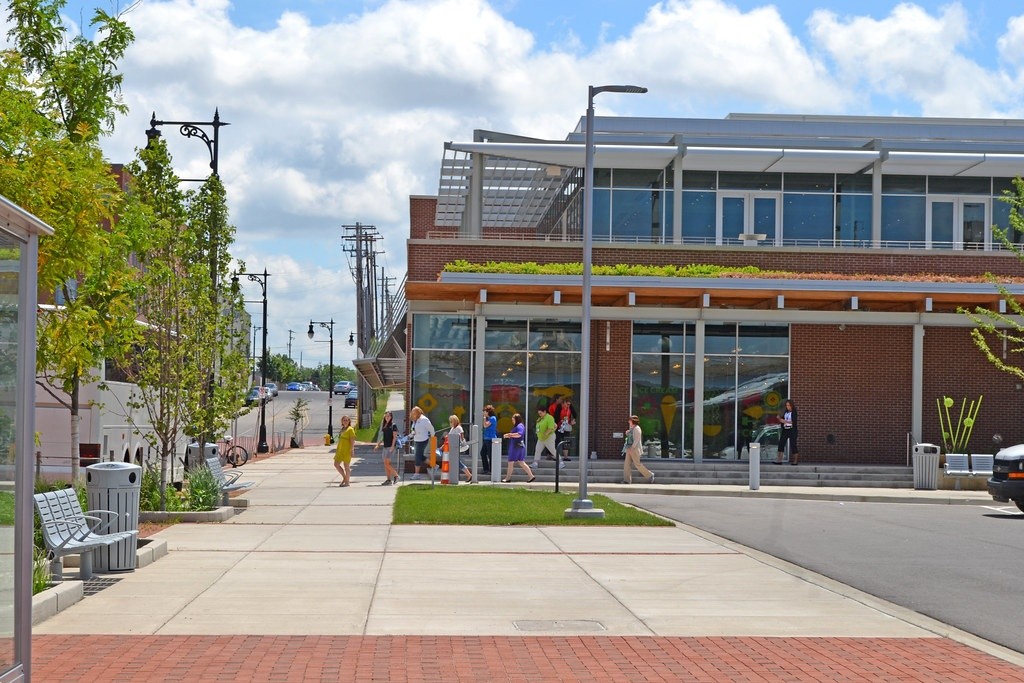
[
  {"xmin": 243, "ymin": 383, "xmax": 279, "ymax": 406},
  {"xmin": 287, "ymin": 381, "xmax": 320, "ymax": 392},
  {"xmin": 718, "ymin": 423, "xmax": 788, "ymax": 460},
  {"xmin": 334, "ymin": 381, "xmax": 358, "ymax": 408},
  {"xmin": 986, "ymin": 444, "xmax": 1024, "ymax": 514}
]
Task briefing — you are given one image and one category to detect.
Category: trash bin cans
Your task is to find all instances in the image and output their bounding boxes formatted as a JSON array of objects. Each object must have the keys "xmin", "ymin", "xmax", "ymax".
[
  {"xmin": 912, "ymin": 443, "xmax": 941, "ymax": 490},
  {"xmin": 187, "ymin": 440, "xmax": 220, "ymax": 467},
  {"xmin": 85, "ymin": 461, "xmax": 144, "ymax": 575}
]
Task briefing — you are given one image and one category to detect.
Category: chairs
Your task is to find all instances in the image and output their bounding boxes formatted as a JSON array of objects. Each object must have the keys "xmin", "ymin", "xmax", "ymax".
[
  {"xmin": 970, "ymin": 454, "xmax": 994, "ymax": 476},
  {"xmin": 943, "ymin": 453, "xmax": 969, "ymax": 489}
]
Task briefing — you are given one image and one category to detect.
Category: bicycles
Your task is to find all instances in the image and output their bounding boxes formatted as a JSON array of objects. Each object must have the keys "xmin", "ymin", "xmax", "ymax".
[{"xmin": 208, "ymin": 436, "xmax": 248, "ymax": 467}]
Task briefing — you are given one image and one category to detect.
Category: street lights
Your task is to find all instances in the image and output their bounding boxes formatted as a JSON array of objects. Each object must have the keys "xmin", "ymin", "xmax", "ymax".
[
  {"xmin": 226, "ymin": 267, "xmax": 272, "ymax": 453},
  {"xmin": 308, "ymin": 317, "xmax": 335, "ymax": 443},
  {"xmin": 562, "ymin": 84, "xmax": 649, "ymax": 519},
  {"xmin": 141, "ymin": 107, "xmax": 230, "ymax": 480}
]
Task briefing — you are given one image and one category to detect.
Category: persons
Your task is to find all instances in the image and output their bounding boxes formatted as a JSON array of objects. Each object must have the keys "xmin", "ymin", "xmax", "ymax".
[
  {"xmin": 547, "ymin": 394, "xmax": 577, "ymax": 461},
  {"xmin": 480, "ymin": 404, "xmax": 497, "ymax": 474},
  {"xmin": 502, "ymin": 413, "xmax": 535, "ymax": 483},
  {"xmin": 407, "ymin": 406, "xmax": 439, "ymax": 480},
  {"xmin": 334, "ymin": 415, "xmax": 357, "ymax": 487},
  {"xmin": 772, "ymin": 399, "xmax": 800, "ymax": 465},
  {"xmin": 439, "ymin": 413, "xmax": 472, "ymax": 483},
  {"xmin": 529, "ymin": 406, "xmax": 566, "ymax": 469},
  {"xmin": 619, "ymin": 415, "xmax": 654, "ymax": 484},
  {"xmin": 373, "ymin": 410, "xmax": 401, "ymax": 486}
]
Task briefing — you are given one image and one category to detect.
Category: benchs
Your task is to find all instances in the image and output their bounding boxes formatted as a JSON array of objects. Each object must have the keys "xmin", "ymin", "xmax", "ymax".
[
  {"xmin": 207, "ymin": 457, "xmax": 255, "ymax": 506},
  {"xmin": 34, "ymin": 488, "xmax": 141, "ymax": 581}
]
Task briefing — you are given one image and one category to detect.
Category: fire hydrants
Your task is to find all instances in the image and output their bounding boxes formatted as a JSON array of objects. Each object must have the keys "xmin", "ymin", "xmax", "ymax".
[{"xmin": 324, "ymin": 434, "xmax": 331, "ymax": 445}]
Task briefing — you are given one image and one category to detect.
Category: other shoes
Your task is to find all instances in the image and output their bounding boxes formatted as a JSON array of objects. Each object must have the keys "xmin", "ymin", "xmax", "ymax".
[
  {"xmin": 559, "ymin": 463, "xmax": 565, "ymax": 469},
  {"xmin": 563, "ymin": 458, "xmax": 571, "ymax": 460},
  {"xmin": 382, "ymin": 480, "xmax": 392, "ymax": 485},
  {"xmin": 465, "ymin": 476, "xmax": 473, "ymax": 482},
  {"xmin": 792, "ymin": 463, "xmax": 798, "ymax": 464},
  {"xmin": 434, "ymin": 465, "xmax": 440, "ymax": 471},
  {"xmin": 527, "ymin": 476, "xmax": 535, "ymax": 482},
  {"xmin": 621, "ymin": 480, "xmax": 630, "ymax": 483},
  {"xmin": 393, "ymin": 475, "xmax": 400, "ymax": 484},
  {"xmin": 772, "ymin": 461, "xmax": 782, "ymax": 465},
  {"xmin": 529, "ymin": 463, "xmax": 538, "ymax": 468},
  {"xmin": 410, "ymin": 473, "xmax": 421, "ymax": 479},
  {"xmin": 501, "ymin": 479, "xmax": 511, "ymax": 482},
  {"xmin": 651, "ymin": 472, "xmax": 654, "ymax": 483}
]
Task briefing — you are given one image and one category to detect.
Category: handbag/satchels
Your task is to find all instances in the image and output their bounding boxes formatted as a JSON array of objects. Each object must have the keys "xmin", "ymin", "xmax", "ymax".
[{"xmin": 460, "ymin": 438, "xmax": 469, "ymax": 452}]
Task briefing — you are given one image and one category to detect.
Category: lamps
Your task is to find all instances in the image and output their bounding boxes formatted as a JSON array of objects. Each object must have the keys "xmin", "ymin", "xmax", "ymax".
[
  {"xmin": 672, "ymin": 361, "xmax": 682, "ymax": 369},
  {"xmin": 839, "ymin": 324, "xmax": 847, "ymax": 330},
  {"xmin": 500, "ymin": 342, "xmax": 549, "ymax": 378},
  {"xmin": 649, "ymin": 369, "xmax": 660, "ymax": 374},
  {"xmin": 704, "ymin": 356, "xmax": 710, "ymax": 363}
]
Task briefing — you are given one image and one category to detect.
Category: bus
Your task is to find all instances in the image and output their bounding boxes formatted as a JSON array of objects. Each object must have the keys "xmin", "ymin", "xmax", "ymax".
[{"xmin": 0, "ymin": 304, "xmax": 214, "ymax": 493}]
